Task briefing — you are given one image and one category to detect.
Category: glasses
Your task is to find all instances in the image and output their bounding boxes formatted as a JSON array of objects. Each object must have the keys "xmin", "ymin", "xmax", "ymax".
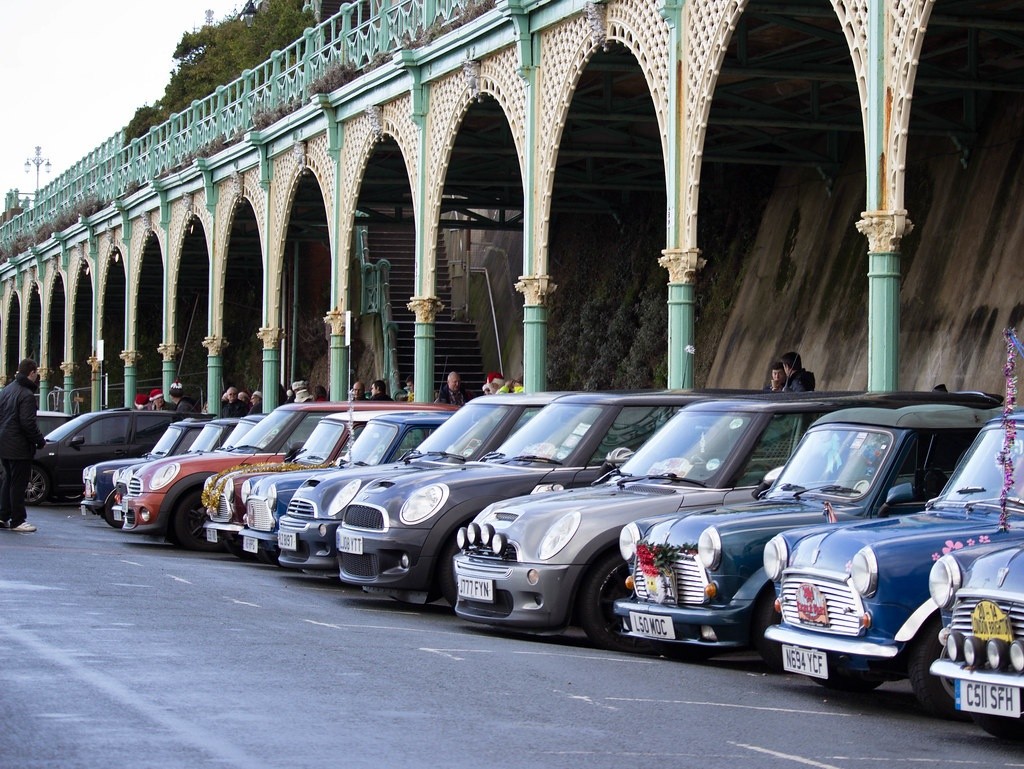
[
  {"xmin": 227, "ymin": 393, "xmax": 237, "ymax": 396},
  {"xmin": 370, "ymin": 387, "xmax": 376, "ymax": 390}
]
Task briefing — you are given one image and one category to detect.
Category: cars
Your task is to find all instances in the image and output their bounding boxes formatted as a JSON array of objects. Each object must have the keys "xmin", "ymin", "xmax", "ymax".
[
  {"xmin": 613, "ymin": 393, "xmax": 1024, "ymax": 672},
  {"xmin": 451, "ymin": 382, "xmax": 1005, "ymax": 654},
  {"xmin": 277, "ymin": 391, "xmax": 457, "ymax": 586},
  {"xmin": 762, "ymin": 412, "xmax": 1024, "ymax": 744},
  {"xmin": 80, "ymin": 417, "xmax": 268, "ymax": 529},
  {"xmin": 238, "ymin": 409, "xmax": 431, "ymax": 570},
  {"xmin": 337, "ymin": 392, "xmax": 727, "ymax": 612},
  {"xmin": 203, "ymin": 411, "xmax": 386, "ymax": 564},
  {"xmin": 111, "ymin": 403, "xmax": 460, "ymax": 553},
  {"xmin": 23, "ymin": 411, "xmax": 214, "ymax": 507}
]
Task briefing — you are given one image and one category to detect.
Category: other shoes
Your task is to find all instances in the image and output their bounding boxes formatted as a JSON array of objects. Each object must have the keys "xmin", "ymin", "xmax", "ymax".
[
  {"xmin": 0, "ymin": 520, "xmax": 10, "ymax": 528},
  {"xmin": 9, "ymin": 522, "xmax": 36, "ymax": 531}
]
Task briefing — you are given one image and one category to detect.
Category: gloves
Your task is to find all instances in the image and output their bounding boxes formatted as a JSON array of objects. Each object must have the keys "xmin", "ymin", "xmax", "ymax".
[{"xmin": 36, "ymin": 438, "xmax": 46, "ymax": 449}]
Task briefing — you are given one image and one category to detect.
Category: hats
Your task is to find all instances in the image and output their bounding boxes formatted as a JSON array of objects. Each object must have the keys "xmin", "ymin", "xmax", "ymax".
[
  {"xmin": 134, "ymin": 394, "xmax": 150, "ymax": 410},
  {"xmin": 149, "ymin": 388, "xmax": 164, "ymax": 401},
  {"xmin": 252, "ymin": 391, "xmax": 263, "ymax": 399},
  {"xmin": 487, "ymin": 372, "xmax": 504, "ymax": 387},
  {"xmin": 168, "ymin": 380, "xmax": 184, "ymax": 398},
  {"xmin": 292, "ymin": 381, "xmax": 307, "ymax": 392},
  {"xmin": 295, "ymin": 389, "xmax": 314, "ymax": 402}
]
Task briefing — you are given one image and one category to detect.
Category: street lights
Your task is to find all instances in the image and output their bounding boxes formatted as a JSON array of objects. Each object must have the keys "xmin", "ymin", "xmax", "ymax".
[{"xmin": 24, "ymin": 146, "xmax": 52, "ymax": 199}]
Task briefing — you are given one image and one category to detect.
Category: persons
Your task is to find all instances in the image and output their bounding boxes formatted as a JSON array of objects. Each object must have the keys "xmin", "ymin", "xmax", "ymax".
[
  {"xmin": 134, "ymin": 372, "xmax": 524, "ymax": 419},
  {"xmin": 762, "ymin": 352, "xmax": 816, "ymax": 393},
  {"xmin": 0, "ymin": 358, "xmax": 45, "ymax": 533}
]
